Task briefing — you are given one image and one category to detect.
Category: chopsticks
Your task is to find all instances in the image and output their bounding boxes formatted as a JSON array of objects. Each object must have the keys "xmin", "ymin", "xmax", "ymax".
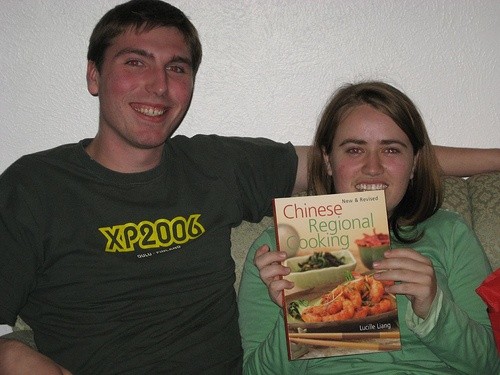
[{"xmin": 288, "ymin": 332, "xmax": 402, "ymax": 350}]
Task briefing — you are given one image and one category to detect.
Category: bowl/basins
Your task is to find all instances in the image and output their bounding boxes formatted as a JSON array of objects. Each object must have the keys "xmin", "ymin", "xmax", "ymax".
[
  {"xmin": 358, "ymin": 245, "xmax": 389, "ymax": 270},
  {"xmin": 284, "ymin": 249, "xmax": 356, "ymax": 289}
]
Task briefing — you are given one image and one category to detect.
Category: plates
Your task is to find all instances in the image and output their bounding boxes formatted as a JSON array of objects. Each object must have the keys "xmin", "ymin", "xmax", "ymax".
[{"xmin": 285, "ymin": 284, "xmax": 398, "ymax": 330}]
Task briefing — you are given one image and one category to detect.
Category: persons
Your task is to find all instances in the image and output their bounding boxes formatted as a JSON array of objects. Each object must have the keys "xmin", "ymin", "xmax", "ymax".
[
  {"xmin": 0, "ymin": 1, "xmax": 500, "ymax": 375},
  {"xmin": 233, "ymin": 81, "xmax": 500, "ymax": 375}
]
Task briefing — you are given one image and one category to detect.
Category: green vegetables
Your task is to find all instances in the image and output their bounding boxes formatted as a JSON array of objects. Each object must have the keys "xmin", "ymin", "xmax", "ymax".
[
  {"xmin": 289, "ymin": 298, "xmax": 310, "ymax": 320},
  {"xmin": 295, "ymin": 249, "xmax": 346, "ymax": 273}
]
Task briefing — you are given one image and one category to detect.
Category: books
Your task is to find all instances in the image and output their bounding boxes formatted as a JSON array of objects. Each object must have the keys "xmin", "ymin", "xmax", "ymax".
[{"xmin": 271, "ymin": 188, "xmax": 403, "ymax": 362}]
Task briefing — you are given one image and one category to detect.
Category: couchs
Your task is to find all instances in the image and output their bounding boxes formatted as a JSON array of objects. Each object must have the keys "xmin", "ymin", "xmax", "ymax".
[{"xmin": 14, "ymin": 171, "xmax": 500, "ymax": 375}]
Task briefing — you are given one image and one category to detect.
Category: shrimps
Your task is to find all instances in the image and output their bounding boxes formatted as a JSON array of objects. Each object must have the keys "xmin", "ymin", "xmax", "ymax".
[{"xmin": 301, "ymin": 275, "xmax": 398, "ymax": 323}]
